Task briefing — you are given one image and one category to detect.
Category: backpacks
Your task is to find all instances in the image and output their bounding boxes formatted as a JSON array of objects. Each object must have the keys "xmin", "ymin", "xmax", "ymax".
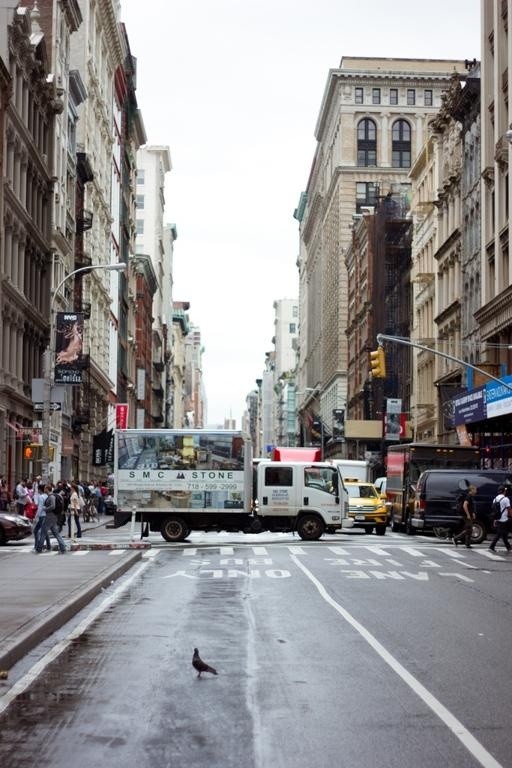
[
  {"xmin": 456, "ymin": 492, "xmax": 467, "ymax": 509},
  {"xmin": 490, "ymin": 496, "xmax": 507, "ymax": 519},
  {"xmin": 45, "ymin": 494, "xmax": 63, "ymax": 515}
]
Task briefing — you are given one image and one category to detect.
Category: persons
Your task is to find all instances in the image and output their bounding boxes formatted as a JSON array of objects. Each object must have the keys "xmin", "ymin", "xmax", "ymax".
[
  {"xmin": 451, "ymin": 484, "xmax": 477, "ymax": 549},
  {"xmin": 1, "ymin": 474, "xmax": 114, "ymax": 556},
  {"xmin": 484, "ymin": 484, "xmax": 512, "ymax": 553}
]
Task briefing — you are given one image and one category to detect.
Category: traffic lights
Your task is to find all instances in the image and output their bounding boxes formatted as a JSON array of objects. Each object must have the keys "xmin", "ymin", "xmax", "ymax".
[
  {"xmin": 369, "ymin": 345, "xmax": 387, "ymax": 379},
  {"xmin": 48, "ymin": 448, "xmax": 55, "ymax": 460},
  {"xmin": 25, "ymin": 445, "xmax": 36, "ymax": 460}
]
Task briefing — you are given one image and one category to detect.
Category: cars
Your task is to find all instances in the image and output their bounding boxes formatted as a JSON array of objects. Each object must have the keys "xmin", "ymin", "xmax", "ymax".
[{"xmin": 0, "ymin": 510, "xmax": 34, "ymax": 547}]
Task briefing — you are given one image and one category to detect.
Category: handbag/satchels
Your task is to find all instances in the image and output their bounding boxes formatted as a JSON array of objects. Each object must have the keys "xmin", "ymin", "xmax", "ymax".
[{"xmin": 78, "ymin": 497, "xmax": 85, "ymax": 508}]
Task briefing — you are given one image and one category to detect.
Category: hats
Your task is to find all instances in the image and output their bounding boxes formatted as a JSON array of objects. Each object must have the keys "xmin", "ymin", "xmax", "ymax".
[{"xmin": 497, "ymin": 485, "xmax": 508, "ymax": 493}]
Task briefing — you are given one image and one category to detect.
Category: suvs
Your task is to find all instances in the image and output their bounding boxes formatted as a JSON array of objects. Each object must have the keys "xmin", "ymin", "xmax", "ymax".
[{"xmin": 322, "ymin": 477, "xmax": 391, "ymax": 538}]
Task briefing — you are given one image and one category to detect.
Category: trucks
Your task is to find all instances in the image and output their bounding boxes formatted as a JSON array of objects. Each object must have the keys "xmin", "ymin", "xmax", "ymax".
[{"xmin": 105, "ymin": 425, "xmax": 354, "ymax": 541}]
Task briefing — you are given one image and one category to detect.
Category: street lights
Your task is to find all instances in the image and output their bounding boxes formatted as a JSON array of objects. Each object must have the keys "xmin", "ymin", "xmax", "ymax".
[
  {"xmin": 293, "ymin": 386, "xmax": 348, "ymax": 408},
  {"xmin": 41, "ymin": 262, "xmax": 129, "ymax": 487}
]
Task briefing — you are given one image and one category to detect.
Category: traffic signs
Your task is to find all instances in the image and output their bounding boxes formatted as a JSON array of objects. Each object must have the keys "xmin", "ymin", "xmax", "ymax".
[{"xmin": 33, "ymin": 401, "xmax": 61, "ymax": 412}]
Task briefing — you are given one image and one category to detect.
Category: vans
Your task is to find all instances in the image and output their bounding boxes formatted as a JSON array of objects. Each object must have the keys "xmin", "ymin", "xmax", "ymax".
[
  {"xmin": 372, "ymin": 476, "xmax": 388, "ymax": 496},
  {"xmin": 408, "ymin": 465, "xmax": 511, "ymax": 545}
]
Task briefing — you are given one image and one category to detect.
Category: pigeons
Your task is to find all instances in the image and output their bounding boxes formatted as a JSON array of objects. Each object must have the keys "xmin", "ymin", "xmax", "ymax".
[{"xmin": 192, "ymin": 647, "xmax": 218, "ymax": 677}]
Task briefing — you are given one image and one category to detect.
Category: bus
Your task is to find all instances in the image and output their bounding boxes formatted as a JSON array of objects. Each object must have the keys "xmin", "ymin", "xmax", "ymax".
[
  {"xmin": 323, "ymin": 457, "xmax": 373, "ymax": 484},
  {"xmin": 380, "ymin": 441, "xmax": 483, "ymax": 537}
]
